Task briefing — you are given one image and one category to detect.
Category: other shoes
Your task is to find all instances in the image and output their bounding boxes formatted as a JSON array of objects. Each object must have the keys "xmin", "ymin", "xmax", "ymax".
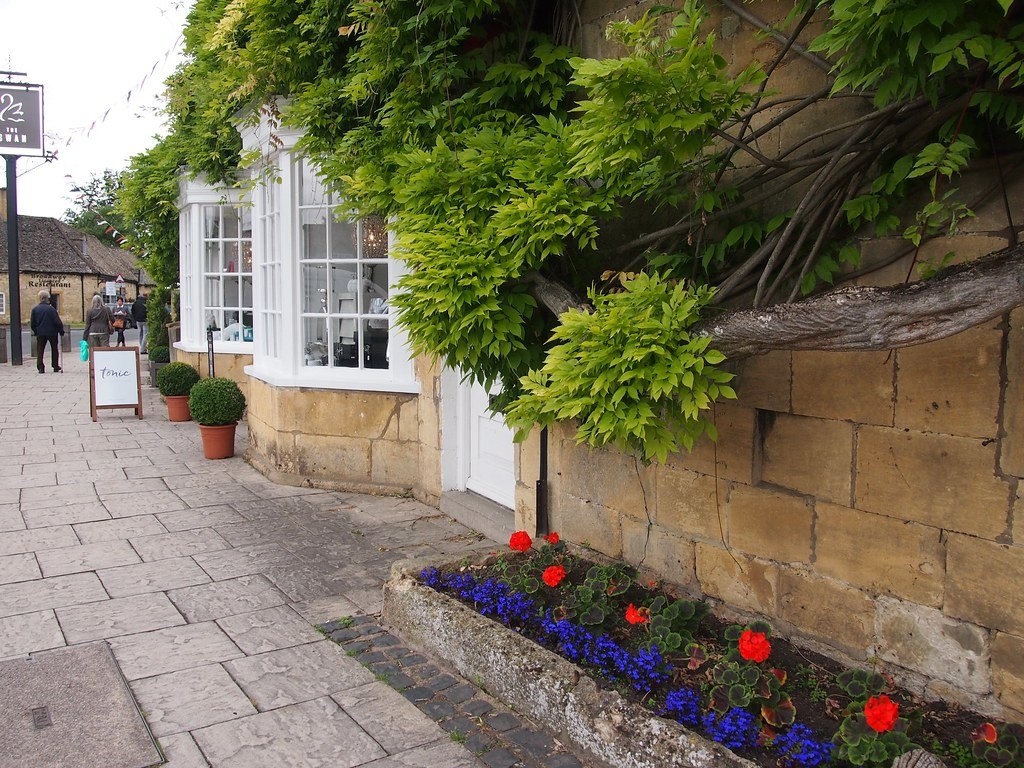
[
  {"xmin": 54, "ymin": 366, "xmax": 61, "ymax": 372},
  {"xmin": 140, "ymin": 351, "xmax": 148, "ymax": 354},
  {"xmin": 39, "ymin": 366, "xmax": 45, "ymax": 373}
]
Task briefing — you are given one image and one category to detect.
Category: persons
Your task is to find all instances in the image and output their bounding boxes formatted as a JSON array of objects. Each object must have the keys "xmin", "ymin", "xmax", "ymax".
[
  {"xmin": 131, "ymin": 294, "xmax": 149, "ymax": 353},
  {"xmin": 111, "ymin": 297, "xmax": 130, "ymax": 347},
  {"xmin": 83, "ymin": 295, "xmax": 115, "ymax": 348},
  {"xmin": 30, "ymin": 290, "xmax": 65, "ymax": 373}
]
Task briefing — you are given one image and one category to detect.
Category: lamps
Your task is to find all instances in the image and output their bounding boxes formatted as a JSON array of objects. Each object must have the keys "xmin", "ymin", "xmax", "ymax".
[
  {"xmin": 350, "ymin": 214, "xmax": 387, "ymax": 268},
  {"xmin": 231, "ymin": 230, "xmax": 252, "ymax": 285}
]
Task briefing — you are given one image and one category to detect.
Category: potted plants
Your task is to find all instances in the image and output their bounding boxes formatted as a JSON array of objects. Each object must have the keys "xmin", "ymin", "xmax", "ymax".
[
  {"xmin": 156, "ymin": 361, "xmax": 201, "ymax": 422},
  {"xmin": 186, "ymin": 377, "xmax": 247, "ymax": 460}
]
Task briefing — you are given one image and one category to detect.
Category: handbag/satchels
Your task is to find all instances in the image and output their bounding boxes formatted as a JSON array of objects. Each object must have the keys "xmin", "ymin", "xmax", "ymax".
[
  {"xmin": 79, "ymin": 340, "xmax": 89, "ymax": 361},
  {"xmin": 113, "ymin": 317, "xmax": 123, "ymax": 328}
]
